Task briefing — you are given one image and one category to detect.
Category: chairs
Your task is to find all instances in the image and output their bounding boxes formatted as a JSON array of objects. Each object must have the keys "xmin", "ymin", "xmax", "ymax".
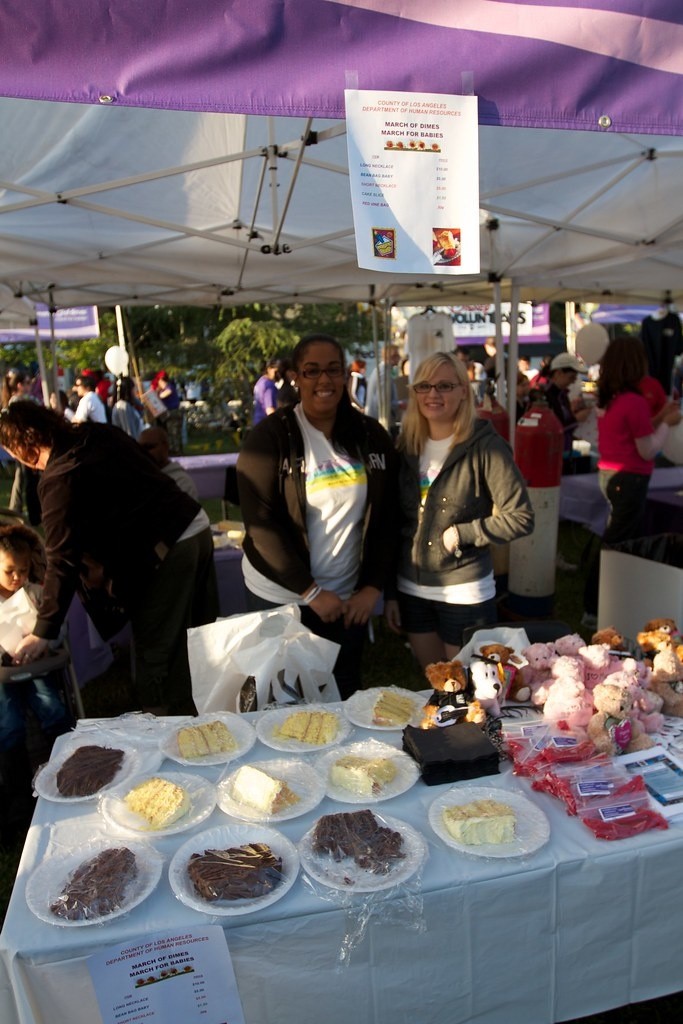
[{"xmin": 0, "ymin": 509, "xmax": 85, "ymax": 726}]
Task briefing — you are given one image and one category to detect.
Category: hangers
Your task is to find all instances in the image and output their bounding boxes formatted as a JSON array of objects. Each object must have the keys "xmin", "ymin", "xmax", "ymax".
[{"xmin": 421, "ymin": 306, "xmax": 436, "ymax": 315}]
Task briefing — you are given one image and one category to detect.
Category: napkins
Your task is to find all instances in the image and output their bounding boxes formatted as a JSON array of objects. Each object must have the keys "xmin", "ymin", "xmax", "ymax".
[{"xmin": 402, "ymin": 722, "xmax": 500, "ymax": 786}]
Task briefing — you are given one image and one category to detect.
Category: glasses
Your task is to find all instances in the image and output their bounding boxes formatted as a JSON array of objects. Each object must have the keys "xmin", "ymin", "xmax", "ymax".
[
  {"xmin": 412, "ymin": 380, "xmax": 461, "ymax": 393},
  {"xmin": 295, "ymin": 364, "xmax": 347, "ymax": 379},
  {"xmin": 22, "ymin": 379, "xmax": 32, "ymax": 386}
]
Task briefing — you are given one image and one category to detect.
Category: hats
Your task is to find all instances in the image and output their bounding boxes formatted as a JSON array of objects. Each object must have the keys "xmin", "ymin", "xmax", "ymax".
[{"xmin": 550, "ymin": 353, "xmax": 590, "ymax": 374}]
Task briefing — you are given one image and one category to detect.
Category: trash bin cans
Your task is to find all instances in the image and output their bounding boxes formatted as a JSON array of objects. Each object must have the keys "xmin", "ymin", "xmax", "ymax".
[{"xmin": 596, "ymin": 530, "xmax": 682, "ymax": 646}]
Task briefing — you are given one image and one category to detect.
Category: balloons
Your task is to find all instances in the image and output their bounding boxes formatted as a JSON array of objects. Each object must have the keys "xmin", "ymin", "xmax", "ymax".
[
  {"xmin": 576, "ymin": 324, "xmax": 610, "ymax": 366},
  {"xmin": 104, "ymin": 345, "xmax": 129, "ymax": 376}
]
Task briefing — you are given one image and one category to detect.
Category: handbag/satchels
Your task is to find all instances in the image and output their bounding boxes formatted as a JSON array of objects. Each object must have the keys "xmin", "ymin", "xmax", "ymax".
[{"xmin": 186, "ymin": 603, "xmax": 342, "ymax": 713}]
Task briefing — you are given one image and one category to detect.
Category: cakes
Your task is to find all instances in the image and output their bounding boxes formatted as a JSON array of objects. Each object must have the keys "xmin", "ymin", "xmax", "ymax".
[{"xmin": 47, "ymin": 688, "xmax": 518, "ymax": 919}]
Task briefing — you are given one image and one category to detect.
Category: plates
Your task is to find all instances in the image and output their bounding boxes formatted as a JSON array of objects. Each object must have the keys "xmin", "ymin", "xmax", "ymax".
[
  {"xmin": 168, "ymin": 822, "xmax": 300, "ymax": 917},
  {"xmin": 429, "ymin": 786, "xmax": 551, "ymax": 858},
  {"xmin": 432, "ymin": 246, "xmax": 461, "ymax": 263},
  {"xmin": 345, "ymin": 686, "xmax": 428, "ymax": 730},
  {"xmin": 24, "ymin": 834, "xmax": 163, "ymax": 927},
  {"xmin": 101, "ymin": 771, "xmax": 217, "ymax": 834},
  {"xmin": 217, "ymin": 758, "xmax": 326, "ymax": 823},
  {"xmin": 34, "ymin": 742, "xmax": 141, "ymax": 803},
  {"xmin": 316, "ymin": 741, "xmax": 421, "ymax": 805},
  {"xmin": 255, "ymin": 706, "xmax": 350, "ymax": 753},
  {"xmin": 297, "ymin": 813, "xmax": 426, "ymax": 892},
  {"xmin": 158, "ymin": 711, "xmax": 256, "ymax": 767}
]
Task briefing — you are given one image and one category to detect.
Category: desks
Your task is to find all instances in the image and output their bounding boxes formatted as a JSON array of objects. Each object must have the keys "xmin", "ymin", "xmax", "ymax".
[
  {"xmin": 561, "ymin": 466, "xmax": 682, "ymax": 565},
  {"xmin": 168, "ymin": 453, "xmax": 241, "ymax": 519},
  {"xmin": 0, "ymin": 690, "xmax": 683, "ymax": 1024}
]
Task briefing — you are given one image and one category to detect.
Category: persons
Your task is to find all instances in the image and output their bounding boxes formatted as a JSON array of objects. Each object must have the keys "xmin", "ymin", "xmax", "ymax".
[
  {"xmin": 581, "ymin": 332, "xmax": 683, "ymax": 633},
  {"xmin": 350, "ymin": 360, "xmax": 368, "ymax": 414},
  {"xmin": 397, "ymin": 351, "xmax": 535, "ymax": 668},
  {"xmin": 235, "ymin": 335, "xmax": 398, "ymax": 698},
  {"xmin": 367, "ymin": 344, "xmax": 409, "ymax": 438},
  {"xmin": 0, "ymin": 362, "xmax": 221, "ymax": 885},
  {"xmin": 456, "ymin": 337, "xmax": 591, "ymax": 453},
  {"xmin": 251, "ymin": 356, "xmax": 298, "ymax": 425}
]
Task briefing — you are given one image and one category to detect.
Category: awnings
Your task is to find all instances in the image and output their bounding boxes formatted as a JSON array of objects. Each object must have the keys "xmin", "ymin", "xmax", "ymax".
[{"xmin": 0, "ymin": 0, "xmax": 683, "ymax": 459}]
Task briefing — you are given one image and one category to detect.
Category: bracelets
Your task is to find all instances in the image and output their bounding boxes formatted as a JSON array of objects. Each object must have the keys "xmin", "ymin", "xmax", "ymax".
[
  {"xmin": 304, "ymin": 585, "xmax": 324, "ymax": 605},
  {"xmin": 449, "ymin": 526, "xmax": 462, "ymax": 559}
]
